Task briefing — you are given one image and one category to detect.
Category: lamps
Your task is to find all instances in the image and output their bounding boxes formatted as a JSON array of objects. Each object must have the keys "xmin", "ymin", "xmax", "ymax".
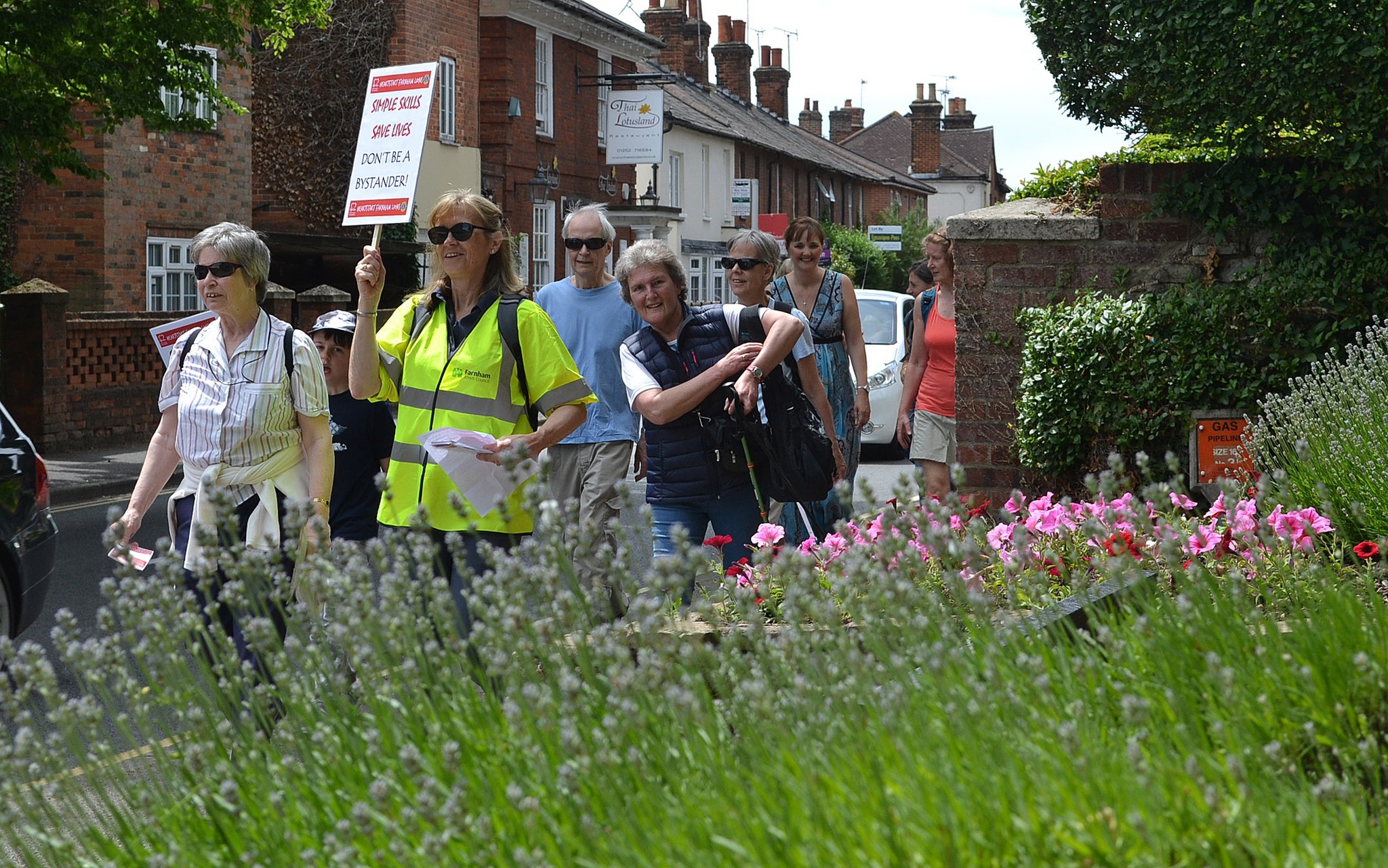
[
  {"xmin": 514, "ymin": 161, "xmax": 553, "ymax": 204},
  {"xmin": 622, "ymin": 180, "xmax": 660, "ymax": 206}
]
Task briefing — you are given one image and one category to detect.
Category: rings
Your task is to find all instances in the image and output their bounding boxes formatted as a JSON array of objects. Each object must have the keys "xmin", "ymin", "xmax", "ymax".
[{"xmin": 742, "ymin": 354, "xmax": 746, "ymax": 361}]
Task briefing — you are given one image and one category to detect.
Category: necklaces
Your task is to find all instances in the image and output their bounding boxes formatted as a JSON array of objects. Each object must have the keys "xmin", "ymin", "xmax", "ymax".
[{"xmin": 793, "ymin": 271, "xmax": 820, "ymax": 307}]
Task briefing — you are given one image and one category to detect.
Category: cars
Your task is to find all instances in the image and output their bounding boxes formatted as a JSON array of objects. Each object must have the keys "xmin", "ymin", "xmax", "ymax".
[
  {"xmin": 0, "ymin": 404, "xmax": 57, "ymax": 654},
  {"xmin": 847, "ymin": 288, "xmax": 924, "ymax": 456}
]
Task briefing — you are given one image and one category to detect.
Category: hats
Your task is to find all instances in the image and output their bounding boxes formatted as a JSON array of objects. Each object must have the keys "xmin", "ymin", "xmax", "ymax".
[{"xmin": 308, "ymin": 310, "xmax": 356, "ymax": 335}]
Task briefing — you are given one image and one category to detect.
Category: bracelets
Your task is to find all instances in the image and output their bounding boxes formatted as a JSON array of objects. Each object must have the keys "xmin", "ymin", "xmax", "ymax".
[{"xmin": 354, "ymin": 308, "xmax": 378, "ymax": 319}]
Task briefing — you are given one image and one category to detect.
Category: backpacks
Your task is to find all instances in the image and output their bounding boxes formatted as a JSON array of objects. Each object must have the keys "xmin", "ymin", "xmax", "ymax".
[{"xmin": 736, "ymin": 302, "xmax": 836, "ymax": 505}]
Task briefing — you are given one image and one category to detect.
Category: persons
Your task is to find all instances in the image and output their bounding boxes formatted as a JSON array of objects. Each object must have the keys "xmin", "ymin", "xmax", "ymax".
[
  {"xmin": 721, "ymin": 229, "xmax": 847, "ymax": 545},
  {"xmin": 307, "ymin": 310, "xmax": 396, "ymax": 542},
  {"xmin": 765, "ymin": 216, "xmax": 871, "ymax": 503},
  {"xmin": 897, "ymin": 228, "xmax": 957, "ymax": 509},
  {"xmin": 349, "ymin": 186, "xmax": 599, "ymax": 692},
  {"xmin": 613, "ymin": 238, "xmax": 805, "ymax": 608},
  {"xmin": 101, "ymin": 221, "xmax": 335, "ymax": 743},
  {"xmin": 532, "ymin": 200, "xmax": 651, "ymax": 627}
]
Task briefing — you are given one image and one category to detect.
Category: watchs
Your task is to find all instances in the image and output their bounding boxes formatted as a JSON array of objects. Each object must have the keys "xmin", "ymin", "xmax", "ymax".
[
  {"xmin": 313, "ymin": 498, "xmax": 330, "ymax": 507},
  {"xmin": 746, "ymin": 365, "xmax": 765, "ymax": 383},
  {"xmin": 856, "ymin": 384, "xmax": 870, "ymax": 392}
]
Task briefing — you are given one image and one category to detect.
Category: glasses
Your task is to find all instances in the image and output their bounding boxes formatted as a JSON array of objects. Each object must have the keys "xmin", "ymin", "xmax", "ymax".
[
  {"xmin": 563, "ymin": 237, "xmax": 608, "ymax": 251},
  {"xmin": 427, "ymin": 222, "xmax": 491, "ymax": 245},
  {"xmin": 193, "ymin": 262, "xmax": 242, "ymax": 280},
  {"xmin": 720, "ymin": 256, "xmax": 767, "ymax": 270}
]
